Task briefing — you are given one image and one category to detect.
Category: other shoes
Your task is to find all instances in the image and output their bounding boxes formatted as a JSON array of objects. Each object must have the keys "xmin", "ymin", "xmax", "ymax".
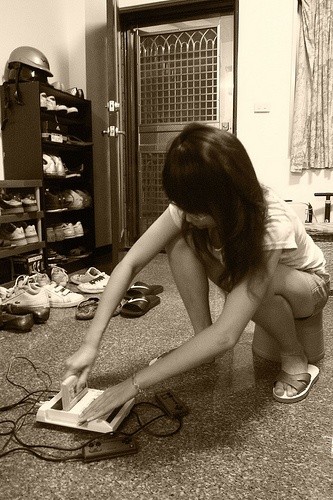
[
  {"xmin": 51, "ymin": 267, "xmax": 69, "ymax": 287},
  {"xmin": 0, "ymin": 273, "xmax": 84, "ymax": 331},
  {"xmin": 0, "ymin": 221, "xmax": 39, "ymax": 248},
  {"xmin": 48, "ymin": 243, "xmax": 93, "ymax": 267},
  {"xmin": 42, "ymin": 132, "xmax": 95, "ymax": 146},
  {"xmin": 40, "ymin": 92, "xmax": 78, "ymax": 119},
  {"xmin": 47, "ymin": 221, "xmax": 83, "ymax": 242},
  {"xmin": 71, "ymin": 267, "xmax": 101, "ymax": 285},
  {"xmin": 0, "ymin": 193, "xmax": 38, "ymax": 214},
  {"xmin": 76, "ymin": 272, "xmax": 110, "ymax": 293},
  {"xmin": 45, "ymin": 189, "xmax": 91, "ymax": 213},
  {"xmin": 42, "ymin": 153, "xmax": 81, "ymax": 178},
  {"xmin": 53, "ymin": 82, "xmax": 84, "ymax": 99},
  {"xmin": 75, "ymin": 296, "xmax": 122, "ymax": 321}
]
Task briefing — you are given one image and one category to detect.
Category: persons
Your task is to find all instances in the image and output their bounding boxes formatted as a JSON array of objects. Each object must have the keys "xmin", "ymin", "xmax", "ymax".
[{"xmin": 59, "ymin": 122, "xmax": 330, "ymax": 426}]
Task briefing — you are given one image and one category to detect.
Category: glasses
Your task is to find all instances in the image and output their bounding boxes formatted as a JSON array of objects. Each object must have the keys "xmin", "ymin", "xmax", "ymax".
[{"xmin": 168, "ymin": 199, "xmax": 207, "ymax": 222}]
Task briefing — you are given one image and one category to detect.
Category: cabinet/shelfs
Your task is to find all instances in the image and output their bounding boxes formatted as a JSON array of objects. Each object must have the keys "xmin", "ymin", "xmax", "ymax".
[
  {"xmin": 0, "ymin": 81, "xmax": 96, "ymax": 274},
  {"xmin": 0, "ymin": 180, "xmax": 46, "ymax": 289}
]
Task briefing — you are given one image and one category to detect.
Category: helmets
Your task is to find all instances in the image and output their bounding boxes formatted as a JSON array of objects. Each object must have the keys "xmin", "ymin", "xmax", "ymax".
[{"xmin": 7, "ymin": 47, "xmax": 54, "ymax": 84}]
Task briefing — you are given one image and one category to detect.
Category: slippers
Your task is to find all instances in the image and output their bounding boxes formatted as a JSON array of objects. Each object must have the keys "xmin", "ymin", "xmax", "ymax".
[
  {"xmin": 123, "ymin": 282, "xmax": 164, "ymax": 299},
  {"xmin": 273, "ymin": 364, "xmax": 320, "ymax": 404},
  {"xmin": 121, "ymin": 294, "xmax": 160, "ymax": 318}
]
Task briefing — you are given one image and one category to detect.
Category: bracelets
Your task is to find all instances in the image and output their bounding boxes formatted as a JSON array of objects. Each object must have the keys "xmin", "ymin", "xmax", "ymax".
[{"xmin": 131, "ymin": 374, "xmax": 143, "ymax": 393}]
